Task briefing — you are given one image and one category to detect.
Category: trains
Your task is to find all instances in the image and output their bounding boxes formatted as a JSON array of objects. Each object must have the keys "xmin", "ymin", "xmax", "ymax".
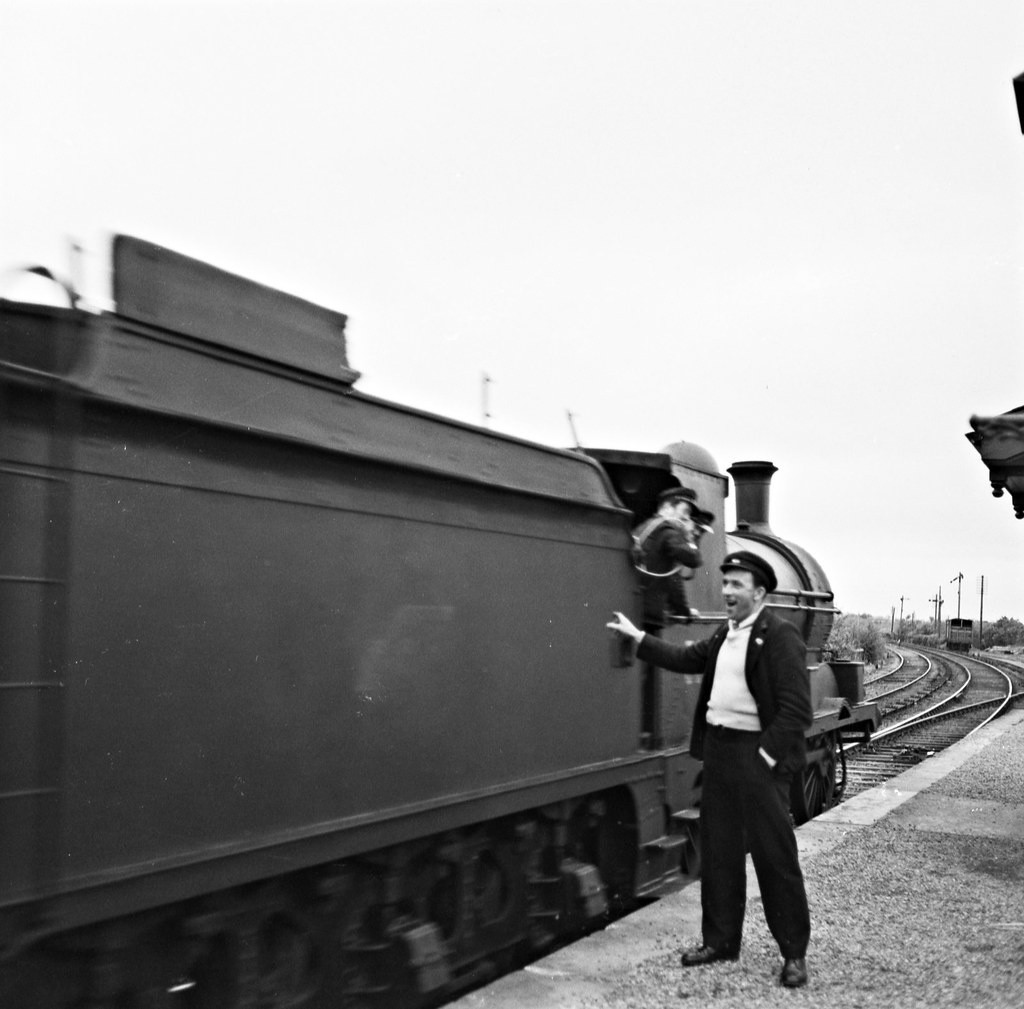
[
  {"xmin": 946, "ymin": 618, "xmax": 974, "ymax": 653},
  {"xmin": 0, "ymin": 236, "xmax": 880, "ymax": 1009}
]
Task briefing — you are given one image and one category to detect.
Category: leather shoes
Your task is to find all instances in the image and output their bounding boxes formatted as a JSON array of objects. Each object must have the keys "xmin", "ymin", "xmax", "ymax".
[
  {"xmin": 782, "ymin": 958, "xmax": 806, "ymax": 986},
  {"xmin": 681, "ymin": 944, "xmax": 740, "ymax": 966}
]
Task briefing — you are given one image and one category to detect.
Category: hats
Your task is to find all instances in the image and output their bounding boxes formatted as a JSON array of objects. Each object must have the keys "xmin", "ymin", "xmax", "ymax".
[{"xmin": 719, "ymin": 551, "xmax": 778, "ymax": 594}]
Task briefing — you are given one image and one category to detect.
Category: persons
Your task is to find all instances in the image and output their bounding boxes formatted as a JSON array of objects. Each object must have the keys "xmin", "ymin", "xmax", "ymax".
[
  {"xmin": 604, "ymin": 550, "xmax": 814, "ymax": 988},
  {"xmin": 632, "ymin": 487, "xmax": 717, "ymax": 751}
]
